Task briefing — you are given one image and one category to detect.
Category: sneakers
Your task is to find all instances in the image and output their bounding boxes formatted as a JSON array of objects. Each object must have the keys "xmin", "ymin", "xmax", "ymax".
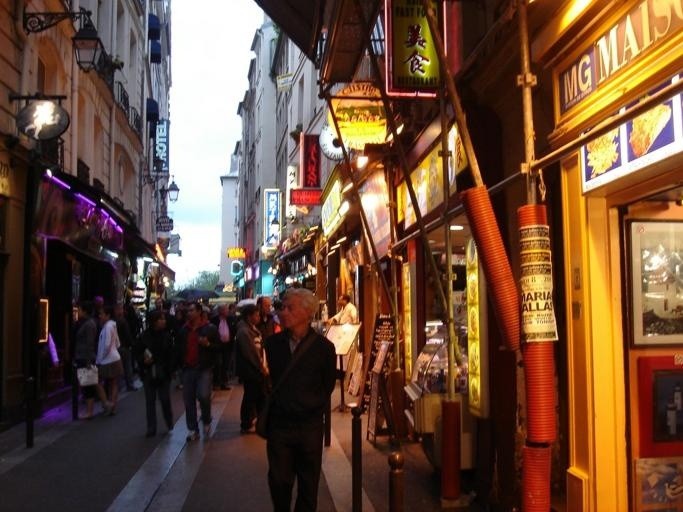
[
  {"xmin": 76, "ymin": 403, "xmax": 118, "ymax": 419},
  {"xmin": 124, "ymin": 383, "xmax": 139, "ymax": 394},
  {"xmin": 239, "ymin": 416, "xmax": 257, "ymax": 434},
  {"xmin": 143, "ymin": 421, "xmax": 213, "ymax": 443}
]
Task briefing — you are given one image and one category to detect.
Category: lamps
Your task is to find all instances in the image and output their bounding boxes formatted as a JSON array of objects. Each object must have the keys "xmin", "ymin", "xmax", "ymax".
[
  {"xmin": 22, "ymin": 5, "xmax": 103, "ymax": 72},
  {"xmin": 145, "ymin": 172, "xmax": 178, "ymax": 202}
]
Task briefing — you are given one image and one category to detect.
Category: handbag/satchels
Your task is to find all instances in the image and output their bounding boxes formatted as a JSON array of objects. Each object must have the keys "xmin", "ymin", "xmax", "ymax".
[{"xmin": 76, "ymin": 367, "xmax": 100, "ymax": 387}]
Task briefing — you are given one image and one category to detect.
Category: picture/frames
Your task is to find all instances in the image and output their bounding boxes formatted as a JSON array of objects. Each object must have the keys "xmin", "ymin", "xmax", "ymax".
[
  {"xmin": 629, "ymin": 218, "xmax": 683, "ymax": 347},
  {"xmin": 635, "ymin": 355, "xmax": 683, "ymax": 511}
]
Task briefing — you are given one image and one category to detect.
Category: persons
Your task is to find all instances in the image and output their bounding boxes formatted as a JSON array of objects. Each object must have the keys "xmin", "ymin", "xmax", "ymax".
[
  {"xmin": 67, "ymin": 295, "xmax": 327, "ymax": 443},
  {"xmin": 254, "ymin": 286, "xmax": 337, "ymax": 511},
  {"xmin": 328, "ymin": 292, "xmax": 361, "ymax": 326}
]
